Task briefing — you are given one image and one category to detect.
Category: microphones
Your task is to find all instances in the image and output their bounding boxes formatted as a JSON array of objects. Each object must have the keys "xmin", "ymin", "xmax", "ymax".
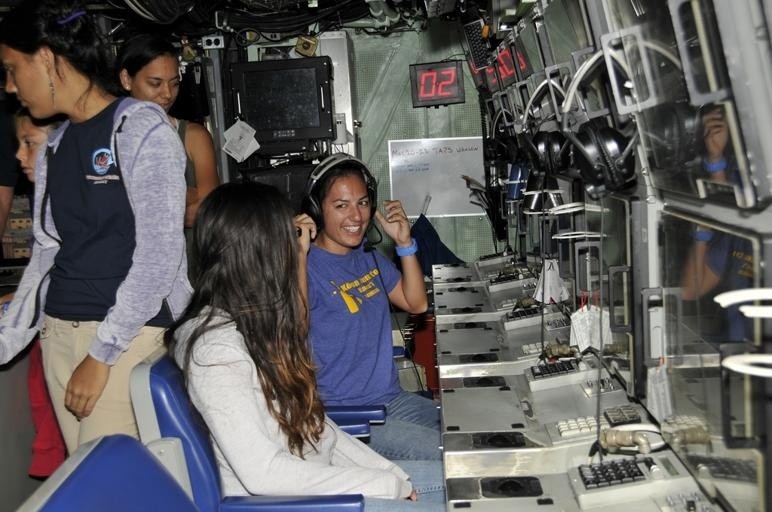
[{"xmin": 363, "ymin": 223, "xmax": 382, "ymax": 247}]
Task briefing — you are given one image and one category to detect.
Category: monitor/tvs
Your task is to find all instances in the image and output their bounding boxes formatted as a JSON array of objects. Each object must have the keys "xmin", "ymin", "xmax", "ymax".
[{"xmin": 231, "ymin": 57, "xmax": 337, "ymax": 142}]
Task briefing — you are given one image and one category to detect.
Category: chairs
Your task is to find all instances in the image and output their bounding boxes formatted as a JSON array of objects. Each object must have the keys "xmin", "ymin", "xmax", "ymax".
[
  {"xmin": 326, "ymin": 404, "xmax": 387, "ymax": 426},
  {"xmin": 130, "ymin": 345, "xmax": 370, "ymax": 511},
  {"xmin": 15, "ymin": 433, "xmax": 201, "ymax": 512}
]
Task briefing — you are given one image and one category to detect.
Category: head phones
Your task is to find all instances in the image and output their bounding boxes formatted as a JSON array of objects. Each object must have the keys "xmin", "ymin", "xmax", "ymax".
[
  {"xmin": 298, "ymin": 152, "xmax": 376, "ymax": 232},
  {"xmin": 491, "ymin": 62, "xmax": 656, "ymax": 199}
]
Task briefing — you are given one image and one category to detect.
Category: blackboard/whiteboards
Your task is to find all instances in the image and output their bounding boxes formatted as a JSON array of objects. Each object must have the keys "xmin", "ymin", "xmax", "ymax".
[{"xmin": 388, "ymin": 135, "xmax": 490, "ymax": 219}]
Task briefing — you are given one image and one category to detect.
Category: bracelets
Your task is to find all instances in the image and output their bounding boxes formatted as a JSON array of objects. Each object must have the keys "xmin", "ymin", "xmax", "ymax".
[{"xmin": 395, "ymin": 237, "xmax": 418, "ymax": 257}]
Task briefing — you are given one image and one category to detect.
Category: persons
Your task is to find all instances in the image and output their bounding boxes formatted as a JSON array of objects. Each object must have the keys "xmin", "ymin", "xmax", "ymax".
[
  {"xmin": 1, "ymin": 98, "xmax": 18, "ymax": 245},
  {"xmin": 290, "ymin": 153, "xmax": 444, "ymax": 460},
  {"xmin": 154, "ymin": 181, "xmax": 448, "ymax": 512},
  {"xmin": 702, "ymin": 107, "xmax": 740, "ymax": 193},
  {"xmin": 1, "ymin": 0, "xmax": 195, "ymax": 458},
  {"xmin": 679, "ymin": 222, "xmax": 754, "ymax": 343},
  {"xmin": 12, "ymin": 105, "xmax": 73, "ymax": 478},
  {"xmin": 116, "ymin": 35, "xmax": 221, "ymax": 292}
]
